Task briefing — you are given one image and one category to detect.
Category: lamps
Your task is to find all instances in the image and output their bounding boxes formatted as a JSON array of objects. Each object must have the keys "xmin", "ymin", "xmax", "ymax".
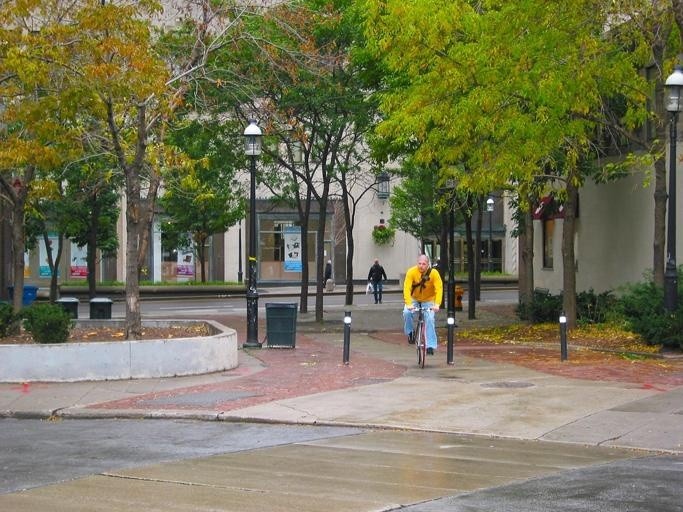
[{"xmin": 375, "ymin": 171, "xmax": 391, "ymax": 199}]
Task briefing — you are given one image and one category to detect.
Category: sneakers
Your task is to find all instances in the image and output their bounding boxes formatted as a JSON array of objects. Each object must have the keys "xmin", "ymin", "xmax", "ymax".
[
  {"xmin": 408, "ymin": 329, "xmax": 415, "ymax": 344},
  {"xmin": 426, "ymin": 348, "xmax": 433, "ymax": 355}
]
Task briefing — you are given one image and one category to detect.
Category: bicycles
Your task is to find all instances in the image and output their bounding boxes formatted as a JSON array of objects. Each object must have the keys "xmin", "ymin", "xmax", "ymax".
[{"xmin": 410, "ymin": 308, "xmax": 439, "ymax": 368}]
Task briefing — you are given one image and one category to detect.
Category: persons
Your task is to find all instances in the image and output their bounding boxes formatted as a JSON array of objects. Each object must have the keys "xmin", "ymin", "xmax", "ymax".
[
  {"xmin": 323, "ymin": 260, "xmax": 331, "ymax": 288},
  {"xmin": 368, "ymin": 259, "xmax": 387, "ymax": 304},
  {"xmin": 402, "ymin": 254, "xmax": 443, "ymax": 355}
]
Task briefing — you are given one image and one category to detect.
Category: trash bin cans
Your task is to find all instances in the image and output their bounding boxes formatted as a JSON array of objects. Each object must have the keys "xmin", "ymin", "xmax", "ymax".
[
  {"xmin": 89, "ymin": 297, "xmax": 114, "ymax": 320},
  {"xmin": 7, "ymin": 285, "xmax": 39, "ymax": 307},
  {"xmin": 55, "ymin": 297, "xmax": 79, "ymax": 319},
  {"xmin": 265, "ymin": 302, "xmax": 298, "ymax": 349}
]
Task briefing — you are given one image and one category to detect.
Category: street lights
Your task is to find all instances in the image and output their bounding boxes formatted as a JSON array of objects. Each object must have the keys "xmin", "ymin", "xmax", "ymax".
[
  {"xmin": 486, "ymin": 197, "xmax": 494, "ymax": 271},
  {"xmin": 243, "ymin": 118, "xmax": 263, "ymax": 350},
  {"xmin": 661, "ymin": 63, "xmax": 683, "ymax": 311}
]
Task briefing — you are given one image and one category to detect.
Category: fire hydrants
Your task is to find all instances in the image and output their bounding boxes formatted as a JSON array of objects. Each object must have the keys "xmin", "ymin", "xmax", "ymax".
[{"xmin": 455, "ymin": 286, "xmax": 464, "ymax": 311}]
